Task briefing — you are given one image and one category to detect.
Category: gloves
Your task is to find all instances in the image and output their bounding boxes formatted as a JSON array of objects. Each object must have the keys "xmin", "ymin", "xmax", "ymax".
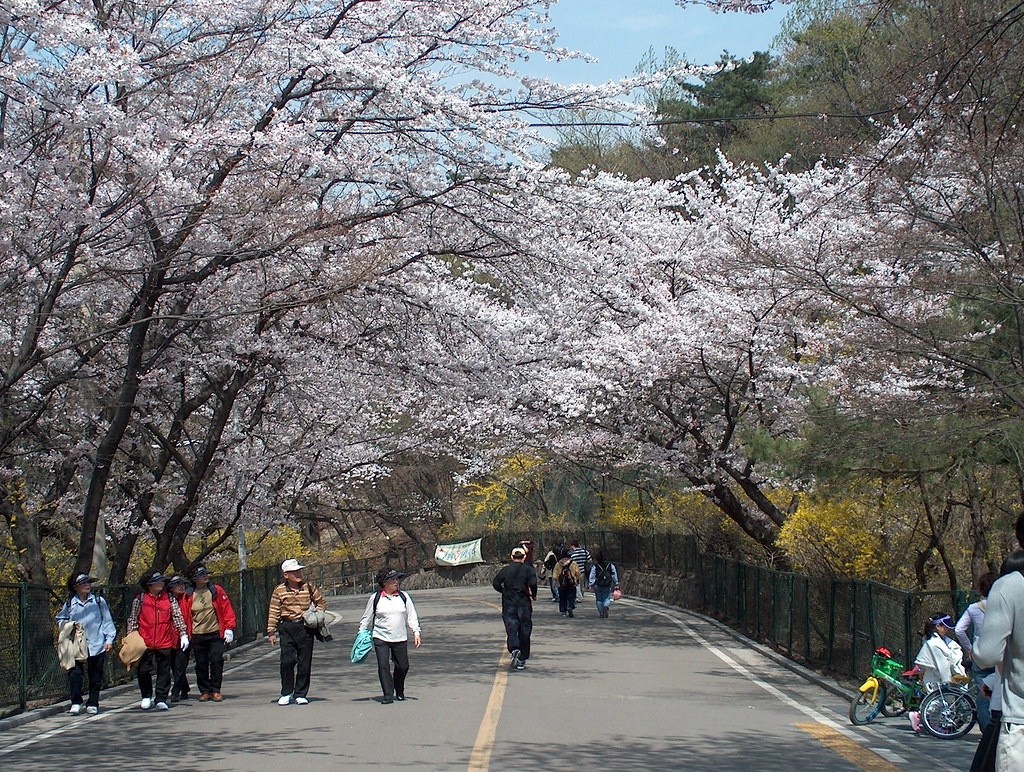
[
  {"xmin": 180, "ymin": 635, "xmax": 189, "ymax": 651},
  {"xmin": 224, "ymin": 629, "xmax": 233, "ymax": 643}
]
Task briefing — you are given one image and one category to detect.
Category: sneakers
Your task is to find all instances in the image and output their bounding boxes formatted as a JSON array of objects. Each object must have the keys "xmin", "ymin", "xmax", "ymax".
[
  {"xmin": 277, "ymin": 691, "xmax": 294, "ymax": 705},
  {"xmin": 155, "ymin": 701, "xmax": 168, "ymax": 710},
  {"xmin": 925, "ymin": 723, "xmax": 949, "ymax": 735},
  {"xmin": 294, "ymin": 697, "xmax": 308, "ymax": 704},
  {"xmin": 141, "ymin": 697, "xmax": 151, "ymax": 709},
  {"xmin": 86, "ymin": 705, "xmax": 98, "ymax": 714},
  {"xmin": 909, "ymin": 711, "xmax": 921, "ymax": 732},
  {"xmin": 70, "ymin": 703, "xmax": 83, "ymax": 713}
]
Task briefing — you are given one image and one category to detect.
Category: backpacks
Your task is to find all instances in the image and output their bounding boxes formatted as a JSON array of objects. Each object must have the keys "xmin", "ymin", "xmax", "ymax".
[{"xmin": 559, "ymin": 559, "xmax": 612, "ymax": 589}]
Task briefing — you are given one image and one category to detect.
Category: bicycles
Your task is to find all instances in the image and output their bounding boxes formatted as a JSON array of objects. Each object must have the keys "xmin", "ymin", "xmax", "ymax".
[{"xmin": 849, "ymin": 648, "xmax": 979, "ymax": 740}]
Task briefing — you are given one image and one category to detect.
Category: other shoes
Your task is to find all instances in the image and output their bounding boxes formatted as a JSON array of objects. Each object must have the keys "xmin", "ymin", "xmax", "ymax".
[
  {"xmin": 179, "ymin": 692, "xmax": 188, "ymax": 700},
  {"xmin": 397, "ymin": 688, "xmax": 403, "ymax": 701},
  {"xmin": 510, "ymin": 598, "xmax": 610, "ymax": 669},
  {"xmin": 211, "ymin": 692, "xmax": 223, "ymax": 702},
  {"xmin": 198, "ymin": 693, "xmax": 212, "ymax": 701},
  {"xmin": 170, "ymin": 694, "xmax": 180, "ymax": 703},
  {"xmin": 381, "ymin": 697, "xmax": 393, "ymax": 704}
]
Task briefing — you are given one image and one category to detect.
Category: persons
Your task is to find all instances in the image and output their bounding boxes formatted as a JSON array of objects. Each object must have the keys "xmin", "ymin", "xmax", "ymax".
[
  {"xmin": 517, "ymin": 538, "xmax": 592, "ymax": 603},
  {"xmin": 267, "ymin": 559, "xmax": 327, "ymax": 705},
  {"xmin": 357, "ymin": 566, "xmax": 421, "ymax": 704},
  {"xmin": 492, "ymin": 548, "xmax": 537, "ymax": 670},
  {"xmin": 127, "ymin": 563, "xmax": 236, "ymax": 711},
  {"xmin": 909, "ymin": 511, "xmax": 1024, "ymax": 772},
  {"xmin": 54, "ymin": 573, "xmax": 116, "ymax": 714},
  {"xmin": 552, "ymin": 549, "xmax": 581, "ymax": 617},
  {"xmin": 588, "ymin": 552, "xmax": 619, "ymax": 618}
]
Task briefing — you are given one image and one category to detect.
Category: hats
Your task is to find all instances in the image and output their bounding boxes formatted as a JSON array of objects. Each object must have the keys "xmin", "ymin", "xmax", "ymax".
[
  {"xmin": 511, "ymin": 547, "xmax": 526, "ymax": 556},
  {"xmin": 382, "ymin": 570, "xmax": 405, "ymax": 582},
  {"xmin": 931, "ymin": 615, "xmax": 956, "ymax": 629},
  {"xmin": 281, "ymin": 558, "xmax": 306, "ymax": 573},
  {"xmin": 72, "ymin": 566, "xmax": 214, "ymax": 589}
]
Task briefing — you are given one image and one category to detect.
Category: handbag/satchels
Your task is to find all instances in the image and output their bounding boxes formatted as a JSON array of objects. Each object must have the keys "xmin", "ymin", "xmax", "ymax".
[{"xmin": 316, "ymin": 624, "xmax": 333, "ymax": 643}]
